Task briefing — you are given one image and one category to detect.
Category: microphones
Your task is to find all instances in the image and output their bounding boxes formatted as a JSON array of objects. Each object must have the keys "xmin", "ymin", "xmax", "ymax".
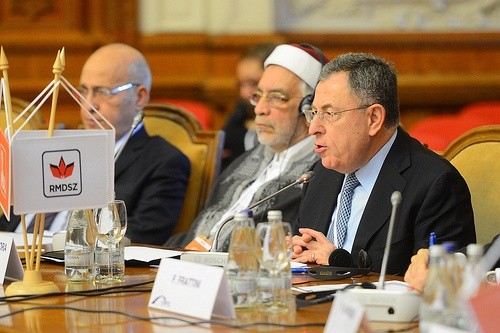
[
  {"xmin": 179, "ymin": 170, "xmax": 316, "ymax": 268},
  {"xmin": 345, "ymin": 191, "xmax": 424, "ymax": 322}
]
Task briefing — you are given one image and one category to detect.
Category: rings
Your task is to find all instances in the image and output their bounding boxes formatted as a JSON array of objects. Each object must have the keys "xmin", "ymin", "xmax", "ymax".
[{"xmin": 312, "ymin": 254, "xmax": 315, "ymax": 260}]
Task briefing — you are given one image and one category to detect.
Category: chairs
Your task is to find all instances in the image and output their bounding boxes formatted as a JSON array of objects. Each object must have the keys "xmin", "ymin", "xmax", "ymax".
[
  {"xmin": 444, "ymin": 125, "xmax": 500, "ymax": 246},
  {"xmin": 0, "ymin": 97, "xmax": 42, "ymax": 135},
  {"xmin": 143, "ymin": 103, "xmax": 226, "ymax": 248}
]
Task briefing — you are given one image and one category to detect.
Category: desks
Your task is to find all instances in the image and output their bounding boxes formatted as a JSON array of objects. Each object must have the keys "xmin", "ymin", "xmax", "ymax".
[{"xmin": 0, "ymin": 243, "xmax": 420, "ymax": 333}]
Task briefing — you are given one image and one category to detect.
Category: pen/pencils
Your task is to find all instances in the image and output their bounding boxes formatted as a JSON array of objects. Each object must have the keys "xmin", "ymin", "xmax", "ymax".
[
  {"xmin": 429, "ymin": 231, "xmax": 436, "ymax": 247},
  {"xmin": 291, "ymin": 268, "xmax": 308, "ymax": 272}
]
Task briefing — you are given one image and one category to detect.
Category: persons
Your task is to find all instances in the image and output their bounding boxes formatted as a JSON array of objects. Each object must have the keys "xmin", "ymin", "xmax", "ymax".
[
  {"xmin": 167, "ymin": 40, "xmax": 500, "ymax": 295},
  {"xmin": 0, "ymin": 43, "xmax": 191, "ymax": 246}
]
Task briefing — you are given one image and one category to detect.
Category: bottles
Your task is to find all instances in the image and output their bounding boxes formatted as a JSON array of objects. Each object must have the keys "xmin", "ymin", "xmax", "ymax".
[
  {"xmin": 257, "ymin": 210, "xmax": 291, "ymax": 308},
  {"xmin": 417, "ymin": 239, "xmax": 484, "ymax": 333},
  {"xmin": 64, "ymin": 208, "xmax": 95, "ymax": 284},
  {"xmin": 226, "ymin": 210, "xmax": 256, "ymax": 312},
  {"xmin": 95, "ymin": 191, "xmax": 125, "ymax": 280}
]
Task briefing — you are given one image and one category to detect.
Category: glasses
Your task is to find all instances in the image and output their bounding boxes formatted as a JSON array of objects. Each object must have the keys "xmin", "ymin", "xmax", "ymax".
[
  {"xmin": 302, "ymin": 105, "xmax": 370, "ymax": 124},
  {"xmin": 76, "ymin": 82, "xmax": 141, "ymax": 101},
  {"xmin": 248, "ymin": 92, "xmax": 304, "ymax": 108}
]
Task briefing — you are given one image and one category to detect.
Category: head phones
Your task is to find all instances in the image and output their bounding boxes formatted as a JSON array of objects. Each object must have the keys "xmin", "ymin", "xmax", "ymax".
[
  {"xmin": 305, "ymin": 248, "xmax": 373, "ymax": 282},
  {"xmin": 295, "ymin": 280, "xmax": 375, "ymax": 306},
  {"xmin": 297, "ymin": 43, "xmax": 327, "ymax": 117}
]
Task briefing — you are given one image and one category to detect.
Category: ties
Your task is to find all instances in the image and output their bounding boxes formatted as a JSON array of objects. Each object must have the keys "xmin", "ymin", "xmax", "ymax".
[{"xmin": 326, "ymin": 173, "xmax": 361, "ymax": 251}]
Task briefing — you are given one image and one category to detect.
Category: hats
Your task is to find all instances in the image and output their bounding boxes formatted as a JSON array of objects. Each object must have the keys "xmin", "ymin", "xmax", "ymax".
[{"xmin": 262, "ymin": 43, "xmax": 331, "ymax": 89}]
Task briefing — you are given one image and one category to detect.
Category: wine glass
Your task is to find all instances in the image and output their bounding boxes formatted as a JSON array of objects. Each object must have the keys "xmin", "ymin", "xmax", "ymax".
[
  {"xmin": 95, "ymin": 200, "xmax": 128, "ymax": 286},
  {"xmin": 254, "ymin": 222, "xmax": 293, "ymax": 312}
]
